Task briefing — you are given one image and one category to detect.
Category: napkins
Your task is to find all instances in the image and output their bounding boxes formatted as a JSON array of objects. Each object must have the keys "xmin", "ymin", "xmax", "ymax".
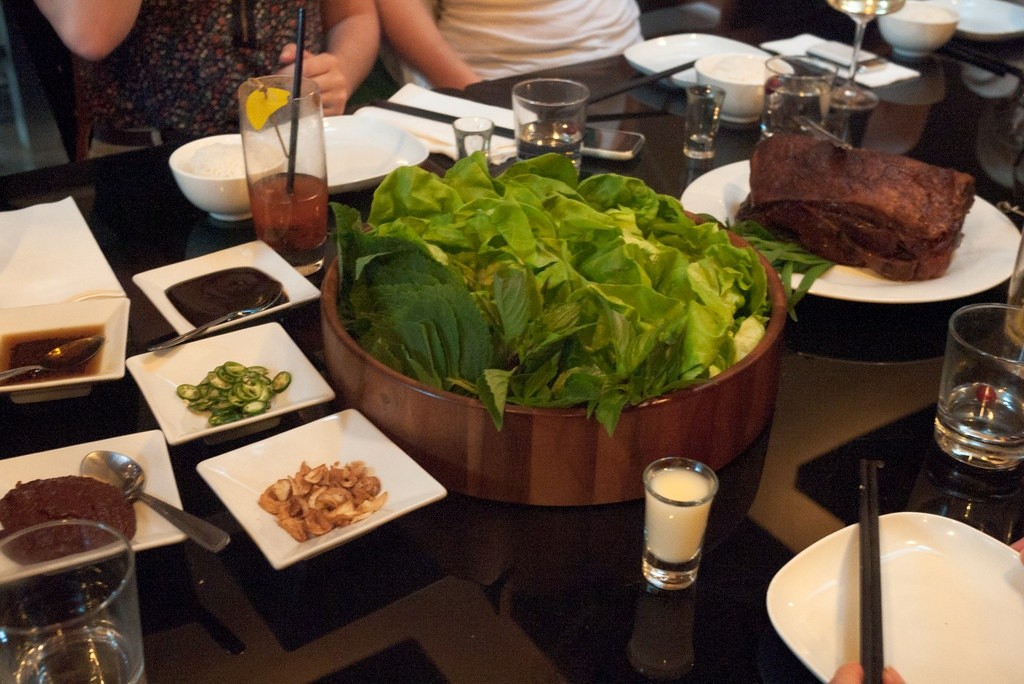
[
  {"xmin": 353, "ymin": 83, "xmax": 517, "ymax": 165},
  {"xmin": 0, "ymin": 196, "xmax": 126, "ymax": 307},
  {"xmin": 760, "ymin": 32, "xmax": 920, "ymax": 88}
]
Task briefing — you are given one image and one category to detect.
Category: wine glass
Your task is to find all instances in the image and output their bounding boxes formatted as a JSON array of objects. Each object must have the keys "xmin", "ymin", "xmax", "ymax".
[{"xmin": 827, "ymin": 0, "xmax": 906, "ymax": 111}]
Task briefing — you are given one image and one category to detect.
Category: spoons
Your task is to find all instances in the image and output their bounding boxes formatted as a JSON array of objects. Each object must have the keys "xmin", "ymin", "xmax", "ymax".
[
  {"xmin": 146, "ymin": 279, "xmax": 283, "ymax": 352},
  {"xmin": 0, "ymin": 335, "xmax": 105, "ymax": 382},
  {"xmin": 80, "ymin": 450, "xmax": 231, "ymax": 554}
]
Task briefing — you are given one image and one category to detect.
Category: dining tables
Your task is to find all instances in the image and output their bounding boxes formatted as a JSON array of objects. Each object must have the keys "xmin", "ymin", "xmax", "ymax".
[{"xmin": 0, "ymin": 0, "xmax": 1024, "ymax": 684}]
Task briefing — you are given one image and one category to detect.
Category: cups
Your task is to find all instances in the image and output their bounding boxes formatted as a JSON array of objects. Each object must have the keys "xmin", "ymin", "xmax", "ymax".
[
  {"xmin": 512, "ymin": 78, "xmax": 590, "ymax": 177},
  {"xmin": 1004, "ymin": 236, "xmax": 1024, "ymax": 351},
  {"xmin": 683, "ymin": 84, "xmax": 725, "ymax": 159},
  {"xmin": 641, "ymin": 456, "xmax": 719, "ymax": 591},
  {"xmin": 0, "ymin": 519, "xmax": 147, "ymax": 684},
  {"xmin": 934, "ymin": 302, "xmax": 1024, "ymax": 469},
  {"xmin": 761, "ymin": 54, "xmax": 839, "ymax": 141},
  {"xmin": 453, "ymin": 116, "xmax": 494, "ymax": 163},
  {"xmin": 237, "ymin": 74, "xmax": 329, "ymax": 253}
]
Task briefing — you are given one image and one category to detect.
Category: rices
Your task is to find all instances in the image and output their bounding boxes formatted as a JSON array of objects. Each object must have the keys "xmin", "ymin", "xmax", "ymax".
[
  {"xmin": 183, "ymin": 142, "xmax": 267, "ymax": 179},
  {"xmin": 889, "ymin": 4, "xmax": 955, "ymax": 23},
  {"xmin": 701, "ymin": 54, "xmax": 786, "ymax": 84}
]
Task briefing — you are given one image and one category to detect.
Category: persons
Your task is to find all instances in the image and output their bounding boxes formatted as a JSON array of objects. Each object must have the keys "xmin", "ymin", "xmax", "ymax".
[
  {"xmin": 374, "ymin": 0, "xmax": 645, "ymax": 89},
  {"xmin": 35, "ymin": 0, "xmax": 381, "ymax": 160}
]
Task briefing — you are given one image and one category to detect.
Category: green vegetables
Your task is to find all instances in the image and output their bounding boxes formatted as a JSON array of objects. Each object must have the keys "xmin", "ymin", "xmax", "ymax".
[{"xmin": 329, "ymin": 152, "xmax": 831, "ymax": 433}]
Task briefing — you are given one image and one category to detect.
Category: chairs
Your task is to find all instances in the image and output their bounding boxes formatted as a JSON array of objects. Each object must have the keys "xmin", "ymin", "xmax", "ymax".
[{"xmin": 0, "ymin": 0, "xmax": 78, "ymax": 164}]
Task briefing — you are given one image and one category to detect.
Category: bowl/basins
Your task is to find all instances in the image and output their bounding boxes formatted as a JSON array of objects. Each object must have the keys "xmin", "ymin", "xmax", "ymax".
[
  {"xmin": 168, "ymin": 134, "xmax": 285, "ymax": 221},
  {"xmin": 694, "ymin": 53, "xmax": 794, "ymax": 123},
  {"xmin": 878, "ymin": 3, "xmax": 960, "ymax": 58},
  {"xmin": 321, "ymin": 211, "xmax": 788, "ymax": 507}
]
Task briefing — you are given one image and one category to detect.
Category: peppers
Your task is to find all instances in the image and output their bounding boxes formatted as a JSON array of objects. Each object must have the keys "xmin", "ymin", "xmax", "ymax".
[{"xmin": 177, "ymin": 361, "xmax": 292, "ymax": 427}]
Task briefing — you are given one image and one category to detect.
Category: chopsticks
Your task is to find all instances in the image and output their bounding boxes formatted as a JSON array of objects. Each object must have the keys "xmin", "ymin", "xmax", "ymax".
[
  {"xmin": 858, "ymin": 458, "xmax": 885, "ymax": 684},
  {"xmin": 367, "ymin": 97, "xmax": 515, "ymax": 140},
  {"xmin": 947, "ymin": 39, "xmax": 1024, "ymax": 78},
  {"xmin": 586, "ymin": 61, "xmax": 695, "ymax": 105}
]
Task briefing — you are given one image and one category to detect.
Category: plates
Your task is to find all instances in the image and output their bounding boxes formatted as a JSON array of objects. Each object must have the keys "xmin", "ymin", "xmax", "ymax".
[
  {"xmin": 0, "ymin": 297, "xmax": 131, "ymax": 404},
  {"xmin": 262, "ymin": 115, "xmax": 430, "ymax": 195},
  {"xmin": 680, "ymin": 159, "xmax": 1023, "ymax": 304},
  {"xmin": 925, "ymin": 0, "xmax": 1024, "ymax": 40},
  {"xmin": 766, "ymin": 511, "xmax": 1024, "ymax": 684},
  {"xmin": 124, "ymin": 321, "xmax": 335, "ymax": 447},
  {"xmin": 0, "ymin": 429, "xmax": 188, "ymax": 586},
  {"xmin": 624, "ymin": 32, "xmax": 795, "ymax": 91},
  {"xmin": 132, "ymin": 238, "xmax": 321, "ymax": 339},
  {"xmin": 354, "ymin": 82, "xmax": 516, "ymax": 166},
  {"xmin": 0, "ymin": 196, "xmax": 127, "ymax": 308},
  {"xmin": 196, "ymin": 407, "xmax": 448, "ymax": 570}
]
